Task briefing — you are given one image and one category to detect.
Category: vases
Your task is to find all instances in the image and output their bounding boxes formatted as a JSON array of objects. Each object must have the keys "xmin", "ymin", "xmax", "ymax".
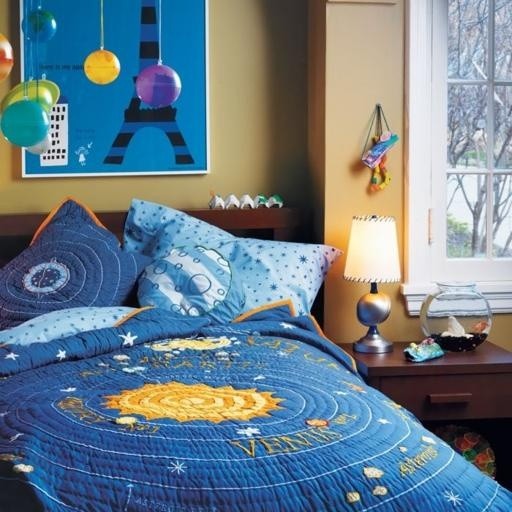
[{"xmin": 419, "ymin": 280, "xmax": 493, "ymax": 353}]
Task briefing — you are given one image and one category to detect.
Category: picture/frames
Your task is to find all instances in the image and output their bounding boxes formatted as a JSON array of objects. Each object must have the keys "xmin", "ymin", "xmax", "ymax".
[{"xmin": 18, "ymin": 0, "xmax": 212, "ymax": 179}]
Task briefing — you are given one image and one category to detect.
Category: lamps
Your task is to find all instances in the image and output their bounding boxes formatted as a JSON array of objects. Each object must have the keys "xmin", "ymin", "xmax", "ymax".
[{"xmin": 341, "ymin": 213, "xmax": 402, "ymax": 354}]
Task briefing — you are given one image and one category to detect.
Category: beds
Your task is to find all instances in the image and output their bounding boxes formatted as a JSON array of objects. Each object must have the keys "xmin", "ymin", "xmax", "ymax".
[{"xmin": 0, "ymin": 208, "xmax": 512, "ymax": 512}]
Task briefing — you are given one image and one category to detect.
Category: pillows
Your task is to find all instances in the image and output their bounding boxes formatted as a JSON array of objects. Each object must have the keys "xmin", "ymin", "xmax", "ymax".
[
  {"xmin": 121, "ymin": 199, "xmax": 343, "ymax": 315},
  {"xmin": 1, "ymin": 193, "xmax": 155, "ymax": 322},
  {"xmin": 135, "ymin": 219, "xmax": 255, "ymax": 326}
]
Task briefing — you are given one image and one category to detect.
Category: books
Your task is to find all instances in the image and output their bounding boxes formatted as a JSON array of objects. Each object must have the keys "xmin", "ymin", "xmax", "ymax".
[{"xmin": 436, "ymin": 269, "xmax": 476, "ymax": 287}]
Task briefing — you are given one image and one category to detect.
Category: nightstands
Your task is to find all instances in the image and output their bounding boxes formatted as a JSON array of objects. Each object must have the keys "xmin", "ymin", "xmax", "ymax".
[{"xmin": 331, "ymin": 338, "xmax": 512, "ymax": 490}]
